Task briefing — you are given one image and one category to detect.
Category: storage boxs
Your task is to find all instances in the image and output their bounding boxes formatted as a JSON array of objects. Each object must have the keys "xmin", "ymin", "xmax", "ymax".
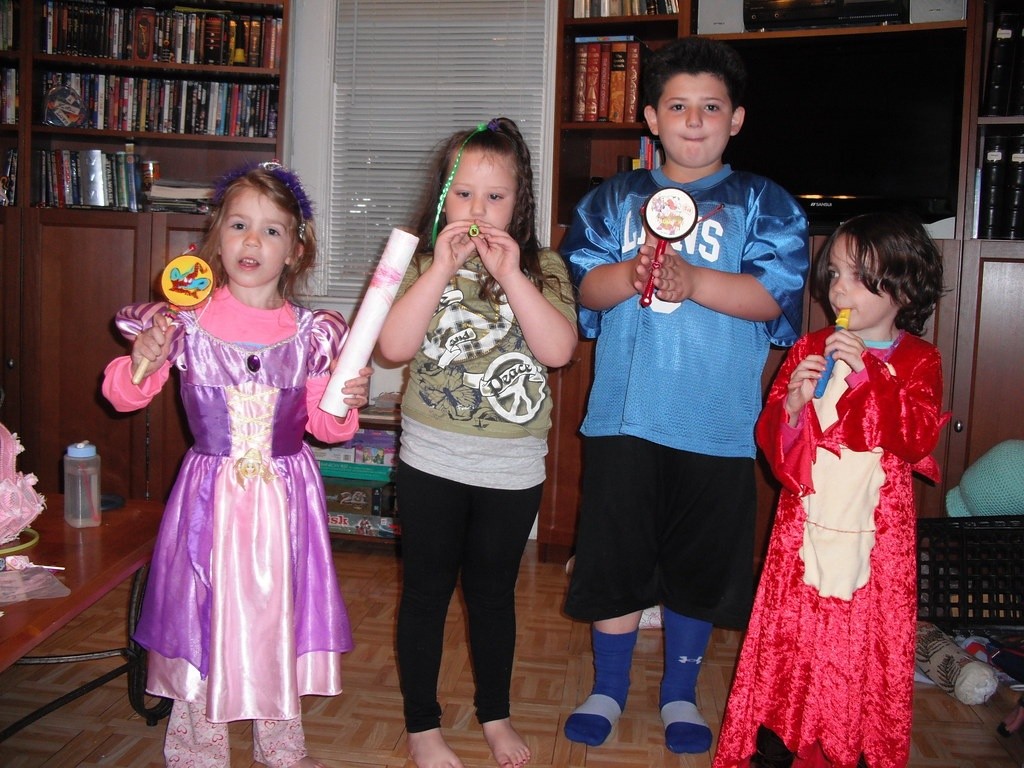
[{"xmin": 917, "ymin": 515, "xmax": 1024, "ymax": 627}]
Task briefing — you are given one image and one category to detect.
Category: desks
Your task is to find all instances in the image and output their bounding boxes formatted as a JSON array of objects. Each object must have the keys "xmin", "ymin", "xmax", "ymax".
[{"xmin": 0, "ymin": 493, "xmax": 174, "ymax": 744}]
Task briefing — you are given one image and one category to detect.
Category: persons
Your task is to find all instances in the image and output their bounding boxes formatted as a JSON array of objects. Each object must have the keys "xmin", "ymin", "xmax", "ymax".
[
  {"xmin": 378, "ymin": 116, "xmax": 578, "ymax": 768},
  {"xmin": 558, "ymin": 35, "xmax": 810, "ymax": 751},
  {"xmin": 102, "ymin": 161, "xmax": 375, "ymax": 768},
  {"xmin": 711, "ymin": 203, "xmax": 949, "ymax": 768}
]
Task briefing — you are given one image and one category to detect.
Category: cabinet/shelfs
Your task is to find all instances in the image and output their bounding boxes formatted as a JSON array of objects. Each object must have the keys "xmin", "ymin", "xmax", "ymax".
[
  {"xmin": 0, "ymin": 0, "xmax": 290, "ymax": 506},
  {"xmin": 329, "ymin": 403, "xmax": 401, "ymax": 544},
  {"xmin": 938, "ymin": 0, "xmax": 1024, "ymax": 520},
  {"xmin": 537, "ymin": 0, "xmax": 975, "ymax": 592}
]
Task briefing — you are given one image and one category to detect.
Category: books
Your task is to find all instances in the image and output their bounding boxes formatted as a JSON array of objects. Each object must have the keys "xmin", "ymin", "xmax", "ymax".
[
  {"xmin": 0, "ymin": 0, "xmax": 282, "ymax": 215},
  {"xmin": 979, "ymin": 10, "xmax": 1024, "ymax": 239},
  {"xmin": 574, "ymin": 0, "xmax": 679, "ymax": 175}
]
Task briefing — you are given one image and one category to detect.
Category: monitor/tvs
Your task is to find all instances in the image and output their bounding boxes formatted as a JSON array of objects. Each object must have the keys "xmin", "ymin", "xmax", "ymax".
[{"xmin": 723, "ymin": 25, "xmax": 967, "ymax": 238}]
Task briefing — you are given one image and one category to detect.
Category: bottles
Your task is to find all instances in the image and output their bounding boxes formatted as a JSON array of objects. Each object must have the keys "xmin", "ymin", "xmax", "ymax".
[{"xmin": 63, "ymin": 444, "xmax": 101, "ymax": 527}]
